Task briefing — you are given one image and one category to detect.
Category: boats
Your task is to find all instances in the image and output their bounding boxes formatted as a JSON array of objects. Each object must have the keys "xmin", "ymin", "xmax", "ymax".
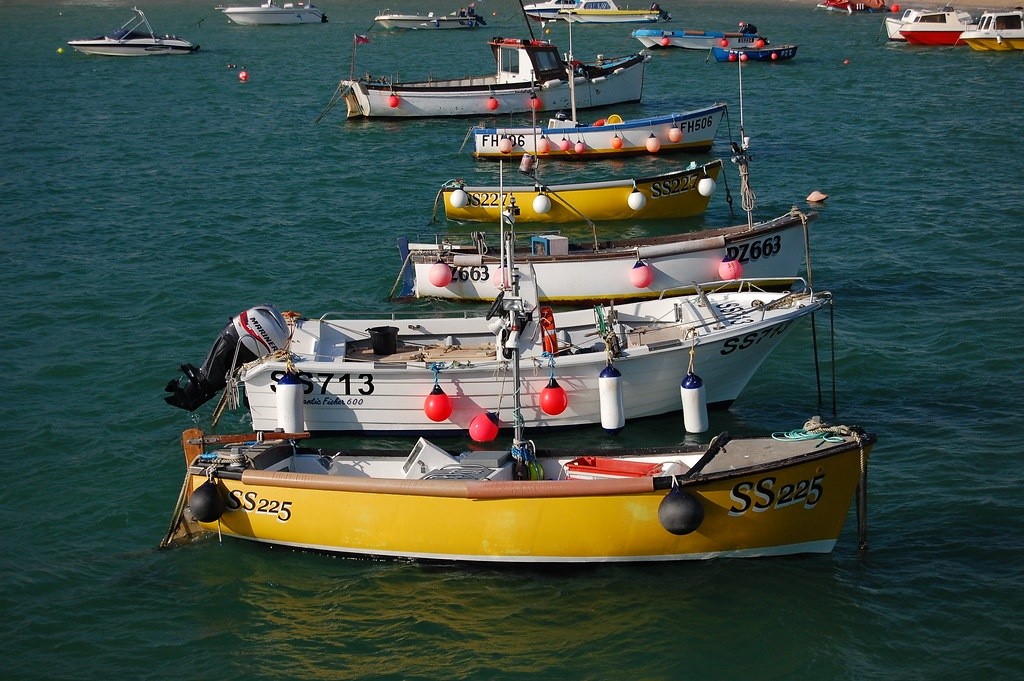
[
  {"xmin": 958, "ymin": 9, "xmax": 1024, "ymax": 51},
  {"xmin": 167, "ymin": 424, "xmax": 867, "ymax": 561},
  {"xmin": 374, "ymin": 14, "xmax": 477, "ymax": 29},
  {"xmin": 522, "ymin": 0, "xmax": 583, "ymax": 19},
  {"xmin": 342, "ymin": 46, "xmax": 648, "ymax": 118},
  {"xmin": 408, "ymin": 209, "xmax": 812, "ymax": 303},
  {"xmin": 339, "ymin": 39, "xmax": 645, "ymax": 119},
  {"xmin": 711, "ymin": 43, "xmax": 797, "ymax": 62},
  {"xmin": 474, "ymin": 102, "xmax": 727, "ymax": 158},
  {"xmin": 884, "ymin": 16, "xmax": 906, "ymax": 40},
  {"xmin": 898, "ymin": 9, "xmax": 973, "ymax": 46},
  {"xmin": 558, "ymin": 1, "xmax": 666, "ymax": 24},
  {"xmin": 67, "ymin": 8, "xmax": 196, "ymax": 55},
  {"xmin": 240, "ymin": 288, "xmax": 834, "ymax": 424},
  {"xmin": 443, "ymin": 159, "xmax": 722, "ymax": 223},
  {"xmin": 632, "ymin": 27, "xmax": 760, "ymax": 51},
  {"xmin": 220, "ymin": 2, "xmax": 326, "ymax": 25}
]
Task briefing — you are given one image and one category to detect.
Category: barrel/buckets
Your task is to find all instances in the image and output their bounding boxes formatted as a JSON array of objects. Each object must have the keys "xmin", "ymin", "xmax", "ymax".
[
  {"xmin": 365, "ymin": 325, "xmax": 399, "ymax": 356},
  {"xmin": 607, "ymin": 115, "xmax": 623, "ymax": 125}
]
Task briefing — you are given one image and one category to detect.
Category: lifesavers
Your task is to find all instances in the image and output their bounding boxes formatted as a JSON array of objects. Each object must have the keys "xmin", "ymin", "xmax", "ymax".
[{"xmin": 541, "ymin": 306, "xmax": 558, "ymax": 357}]
